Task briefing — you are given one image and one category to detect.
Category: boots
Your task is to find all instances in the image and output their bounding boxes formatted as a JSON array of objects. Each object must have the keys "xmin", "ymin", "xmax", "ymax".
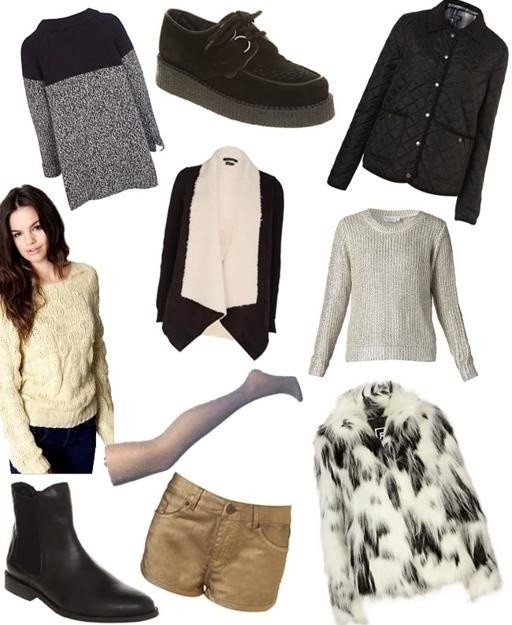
[{"xmin": 2, "ymin": 480, "xmax": 161, "ymax": 625}]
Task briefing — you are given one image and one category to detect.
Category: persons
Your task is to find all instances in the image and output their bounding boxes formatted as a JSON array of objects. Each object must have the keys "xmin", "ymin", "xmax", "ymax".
[
  {"xmin": 107, "ymin": 366, "xmax": 301, "ymax": 487},
  {"xmin": 1, "ymin": 183, "xmax": 117, "ymax": 479}
]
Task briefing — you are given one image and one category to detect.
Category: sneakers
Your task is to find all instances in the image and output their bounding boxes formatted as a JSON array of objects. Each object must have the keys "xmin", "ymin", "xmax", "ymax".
[{"xmin": 150, "ymin": 2, "xmax": 338, "ymax": 128}]
